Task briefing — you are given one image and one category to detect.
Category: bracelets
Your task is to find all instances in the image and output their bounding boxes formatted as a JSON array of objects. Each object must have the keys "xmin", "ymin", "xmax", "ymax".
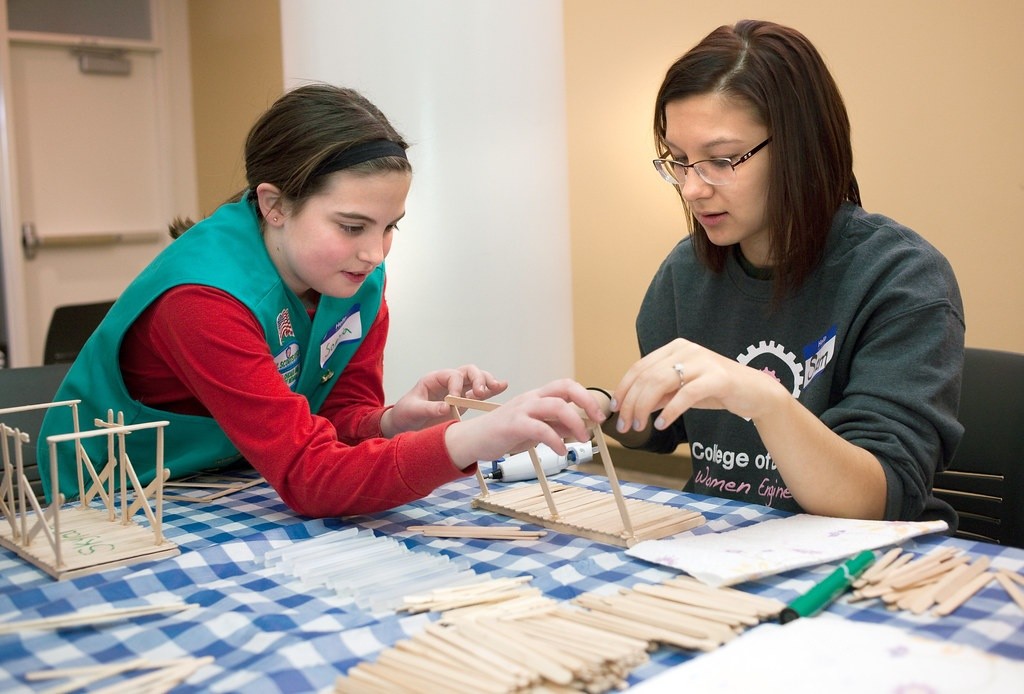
[{"xmin": 586, "ymin": 386, "xmax": 616, "ymax": 421}]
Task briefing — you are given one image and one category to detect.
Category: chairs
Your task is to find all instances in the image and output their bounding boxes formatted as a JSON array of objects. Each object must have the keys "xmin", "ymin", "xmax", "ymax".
[
  {"xmin": 1, "ymin": 299, "xmax": 113, "ymax": 514},
  {"xmin": 932, "ymin": 346, "xmax": 1024, "ymax": 548}
]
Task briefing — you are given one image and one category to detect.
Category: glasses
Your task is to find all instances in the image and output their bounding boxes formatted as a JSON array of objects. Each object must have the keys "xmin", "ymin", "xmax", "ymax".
[{"xmin": 653, "ymin": 136, "xmax": 772, "ymax": 187}]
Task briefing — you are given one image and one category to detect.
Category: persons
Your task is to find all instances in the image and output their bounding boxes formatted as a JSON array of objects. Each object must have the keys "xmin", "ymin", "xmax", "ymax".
[
  {"xmin": 568, "ymin": 18, "xmax": 968, "ymax": 521},
  {"xmin": 36, "ymin": 83, "xmax": 608, "ymax": 518}
]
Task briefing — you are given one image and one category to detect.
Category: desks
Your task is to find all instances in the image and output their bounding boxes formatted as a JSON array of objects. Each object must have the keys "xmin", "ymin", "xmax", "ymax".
[{"xmin": 0, "ymin": 459, "xmax": 1024, "ymax": 694}]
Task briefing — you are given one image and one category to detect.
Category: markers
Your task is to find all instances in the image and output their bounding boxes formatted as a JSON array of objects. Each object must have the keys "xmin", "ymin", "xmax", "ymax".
[{"xmin": 779, "ymin": 550, "xmax": 875, "ymax": 624}]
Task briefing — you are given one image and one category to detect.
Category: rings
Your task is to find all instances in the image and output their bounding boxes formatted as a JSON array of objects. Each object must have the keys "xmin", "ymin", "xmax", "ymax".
[{"xmin": 674, "ymin": 361, "xmax": 686, "ymax": 385}]
